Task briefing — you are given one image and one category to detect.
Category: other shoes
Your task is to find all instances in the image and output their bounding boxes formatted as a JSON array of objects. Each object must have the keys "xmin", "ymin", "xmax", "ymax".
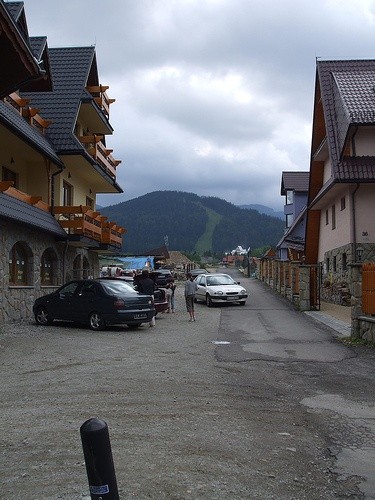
[
  {"xmin": 166, "ymin": 309, "xmax": 175, "ymax": 313},
  {"xmin": 188, "ymin": 316, "xmax": 197, "ymax": 322},
  {"xmin": 148, "ymin": 322, "xmax": 155, "ymax": 327}
]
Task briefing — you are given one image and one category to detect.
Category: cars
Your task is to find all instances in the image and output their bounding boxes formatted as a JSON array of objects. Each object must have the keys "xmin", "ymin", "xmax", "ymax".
[
  {"xmin": 188, "ymin": 269, "xmax": 248, "ymax": 307},
  {"xmin": 33, "ymin": 270, "xmax": 175, "ymax": 332}
]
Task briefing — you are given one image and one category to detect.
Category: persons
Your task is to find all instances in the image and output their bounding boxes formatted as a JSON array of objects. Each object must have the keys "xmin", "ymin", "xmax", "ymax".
[
  {"xmin": 184, "ymin": 272, "xmax": 202, "ymax": 323},
  {"xmin": 167, "ymin": 282, "xmax": 177, "ymax": 314},
  {"xmin": 83, "ymin": 127, "xmax": 93, "ymax": 150},
  {"xmin": 162, "ymin": 284, "xmax": 174, "ymax": 314},
  {"xmin": 135, "ymin": 268, "xmax": 157, "ymax": 327}
]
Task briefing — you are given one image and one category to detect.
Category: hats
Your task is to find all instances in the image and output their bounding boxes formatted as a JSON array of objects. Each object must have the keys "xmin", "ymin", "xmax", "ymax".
[{"xmin": 184, "ymin": 272, "xmax": 192, "ymax": 276}]
